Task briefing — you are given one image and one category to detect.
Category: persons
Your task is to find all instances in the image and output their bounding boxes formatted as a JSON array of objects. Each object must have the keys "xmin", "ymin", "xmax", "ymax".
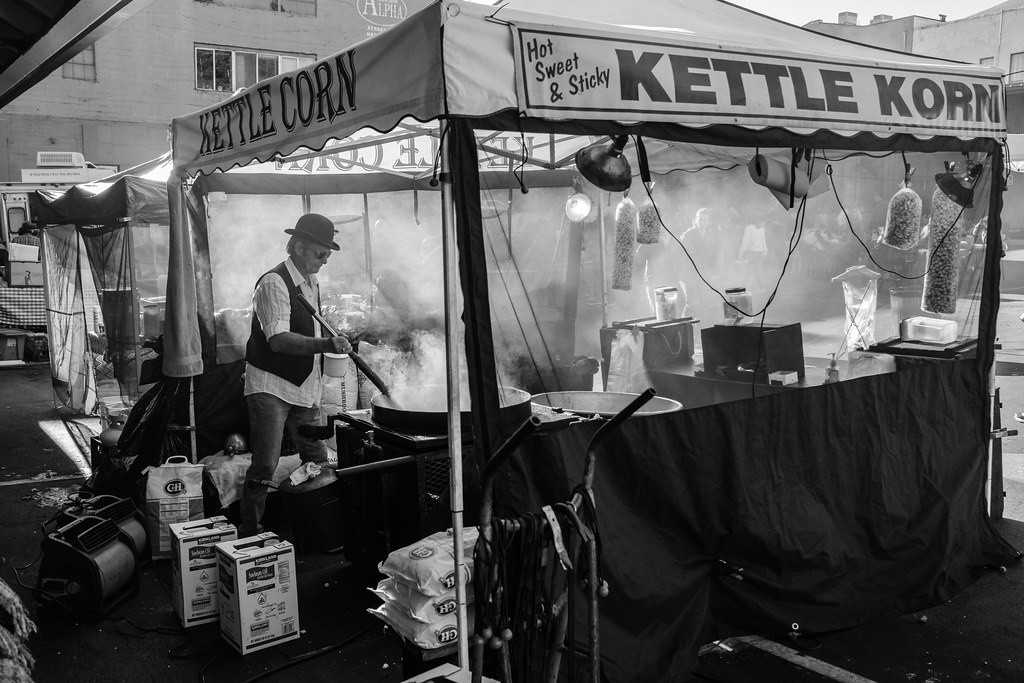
[
  {"xmin": 236, "ymin": 214, "xmax": 353, "ymax": 536},
  {"xmin": 634, "ymin": 191, "xmax": 887, "ymax": 318}
]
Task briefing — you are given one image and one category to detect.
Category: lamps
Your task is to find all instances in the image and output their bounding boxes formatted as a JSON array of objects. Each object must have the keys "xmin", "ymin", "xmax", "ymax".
[
  {"xmin": 935, "ymin": 152, "xmax": 984, "ymax": 209},
  {"xmin": 575, "ymin": 134, "xmax": 633, "ymax": 192},
  {"xmin": 566, "ymin": 183, "xmax": 600, "ymax": 224}
]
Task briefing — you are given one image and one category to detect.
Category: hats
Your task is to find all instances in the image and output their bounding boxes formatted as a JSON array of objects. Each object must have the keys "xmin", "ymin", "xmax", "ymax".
[{"xmin": 284, "ymin": 213, "xmax": 340, "ymax": 250}]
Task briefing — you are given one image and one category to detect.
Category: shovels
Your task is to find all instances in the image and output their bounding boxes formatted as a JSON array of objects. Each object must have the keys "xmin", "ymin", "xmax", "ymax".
[{"xmin": 276, "ymin": 454, "xmax": 415, "ymax": 495}]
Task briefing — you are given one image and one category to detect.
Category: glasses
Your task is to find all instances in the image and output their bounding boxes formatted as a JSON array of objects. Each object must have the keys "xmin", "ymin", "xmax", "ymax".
[{"xmin": 301, "ymin": 241, "xmax": 331, "ymax": 258}]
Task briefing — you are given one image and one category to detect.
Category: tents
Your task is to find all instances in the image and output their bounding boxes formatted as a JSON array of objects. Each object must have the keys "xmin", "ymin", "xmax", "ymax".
[
  {"xmin": 167, "ymin": 0, "xmax": 1007, "ymax": 670},
  {"xmin": 35, "ymin": 150, "xmax": 200, "ymax": 409}
]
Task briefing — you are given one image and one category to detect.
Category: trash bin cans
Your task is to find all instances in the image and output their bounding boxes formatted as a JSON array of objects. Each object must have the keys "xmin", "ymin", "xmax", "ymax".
[{"xmin": 0, "ymin": 328, "xmax": 28, "ymax": 360}]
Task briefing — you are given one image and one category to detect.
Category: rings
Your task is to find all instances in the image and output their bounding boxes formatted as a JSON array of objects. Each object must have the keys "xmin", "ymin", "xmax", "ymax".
[{"xmin": 343, "ymin": 348, "xmax": 345, "ymax": 351}]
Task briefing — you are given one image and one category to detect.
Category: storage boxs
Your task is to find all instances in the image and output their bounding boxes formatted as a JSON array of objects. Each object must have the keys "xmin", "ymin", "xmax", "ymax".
[
  {"xmin": 215, "ymin": 532, "xmax": 300, "ymax": 656},
  {"xmin": 169, "ymin": 516, "xmax": 238, "ymax": 629},
  {"xmin": 7, "ymin": 263, "xmax": 44, "ymax": 287}
]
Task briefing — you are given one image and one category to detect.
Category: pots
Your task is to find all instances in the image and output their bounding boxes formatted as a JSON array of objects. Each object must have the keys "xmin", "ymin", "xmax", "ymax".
[
  {"xmin": 370, "ymin": 382, "xmax": 532, "ymax": 436},
  {"xmin": 530, "ymin": 391, "xmax": 684, "ymax": 418}
]
